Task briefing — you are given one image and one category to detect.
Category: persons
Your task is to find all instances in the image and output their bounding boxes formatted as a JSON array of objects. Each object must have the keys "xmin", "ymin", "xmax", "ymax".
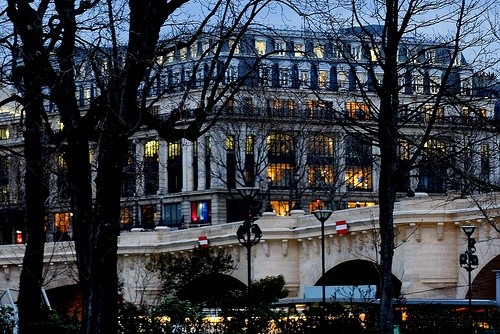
[{"xmin": 407, "ymin": 186, "xmax": 415, "ymax": 198}]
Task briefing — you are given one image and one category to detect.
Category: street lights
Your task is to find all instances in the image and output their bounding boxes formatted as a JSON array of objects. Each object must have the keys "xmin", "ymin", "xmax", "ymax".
[
  {"xmin": 458, "ymin": 225, "xmax": 480, "ymax": 306},
  {"xmin": 309, "ymin": 209, "xmax": 335, "ymax": 309},
  {"xmin": 243, "ymin": 217, "xmax": 260, "ymax": 288}
]
